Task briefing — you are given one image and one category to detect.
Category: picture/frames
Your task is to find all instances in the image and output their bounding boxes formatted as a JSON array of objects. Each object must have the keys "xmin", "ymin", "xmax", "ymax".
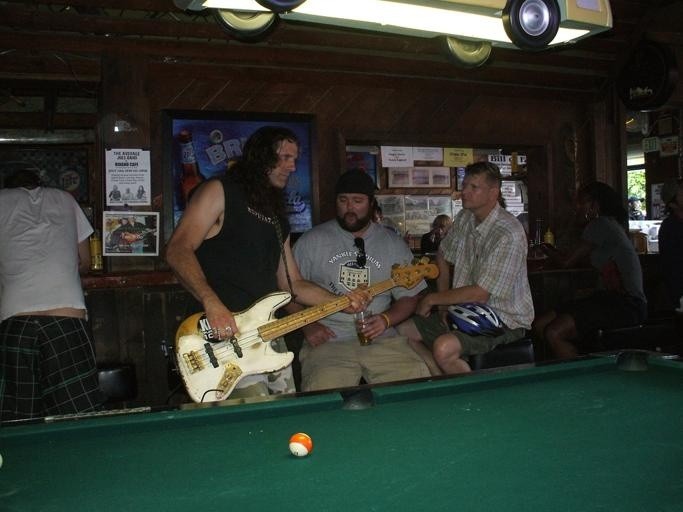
[
  {"xmin": 159, "ymin": 105, "xmax": 324, "ymax": 248},
  {"xmin": 99, "ymin": 209, "xmax": 160, "ymax": 258}
]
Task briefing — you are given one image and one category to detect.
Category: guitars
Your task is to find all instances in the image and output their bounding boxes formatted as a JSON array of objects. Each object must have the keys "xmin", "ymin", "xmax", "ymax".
[{"xmin": 176, "ymin": 258, "xmax": 440, "ymax": 404}]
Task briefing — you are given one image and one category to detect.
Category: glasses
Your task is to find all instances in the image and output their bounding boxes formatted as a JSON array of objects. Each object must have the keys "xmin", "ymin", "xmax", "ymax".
[{"xmin": 353, "ymin": 237, "xmax": 366, "ymax": 266}]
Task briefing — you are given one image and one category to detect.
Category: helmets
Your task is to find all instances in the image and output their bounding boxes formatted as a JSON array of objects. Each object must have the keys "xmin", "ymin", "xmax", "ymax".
[{"xmin": 447, "ymin": 302, "xmax": 502, "ymax": 335}]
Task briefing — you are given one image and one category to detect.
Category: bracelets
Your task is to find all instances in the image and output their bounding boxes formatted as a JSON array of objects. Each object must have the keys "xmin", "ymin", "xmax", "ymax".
[{"xmin": 382, "ymin": 313, "xmax": 389, "ymax": 328}]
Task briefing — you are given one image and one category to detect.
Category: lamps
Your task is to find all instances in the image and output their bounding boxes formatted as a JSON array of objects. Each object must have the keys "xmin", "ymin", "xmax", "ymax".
[{"xmin": 176, "ymin": 0, "xmax": 615, "ymax": 66}]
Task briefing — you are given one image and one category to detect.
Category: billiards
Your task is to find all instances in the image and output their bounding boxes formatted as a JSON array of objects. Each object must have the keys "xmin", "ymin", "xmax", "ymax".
[{"xmin": 289, "ymin": 433, "xmax": 312, "ymax": 456}]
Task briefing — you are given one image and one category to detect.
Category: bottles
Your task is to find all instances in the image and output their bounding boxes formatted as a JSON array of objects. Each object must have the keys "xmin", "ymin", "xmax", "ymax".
[
  {"xmin": 90, "ymin": 226, "xmax": 102, "ymax": 270},
  {"xmin": 544, "ymin": 227, "xmax": 553, "ymax": 255},
  {"xmin": 528, "ymin": 239, "xmax": 535, "ymax": 248},
  {"xmin": 176, "ymin": 127, "xmax": 207, "ymax": 208}
]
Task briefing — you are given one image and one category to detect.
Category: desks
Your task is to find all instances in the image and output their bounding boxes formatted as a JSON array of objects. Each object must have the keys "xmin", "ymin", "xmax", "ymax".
[{"xmin": 0, "ymin": 346, "xmax": 681, "ymax": 512}]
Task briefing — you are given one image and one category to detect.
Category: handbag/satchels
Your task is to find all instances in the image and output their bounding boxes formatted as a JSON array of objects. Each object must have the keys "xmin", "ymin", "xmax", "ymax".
[{"xmin": 601, "ymin": 263, "xmax": 620, "ymax": 290}]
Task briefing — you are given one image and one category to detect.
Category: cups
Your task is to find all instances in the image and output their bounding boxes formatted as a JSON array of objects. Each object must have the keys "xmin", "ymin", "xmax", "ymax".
[{"xmin": 354, "ymin": 306, "xmax": 372, "ymax": 347}]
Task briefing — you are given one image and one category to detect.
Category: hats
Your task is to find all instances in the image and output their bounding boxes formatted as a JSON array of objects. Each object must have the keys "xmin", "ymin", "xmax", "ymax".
[
  {"xmin": 660, "ymin": 178, "xmax": 680, "ymax": 214},
  {"xmin": 336, "ymin": 168, "xmax": 375, "ymax": 195},
  {"xmin": 629, "ymin": 196, "xmax": 644, "ymax": 202}
]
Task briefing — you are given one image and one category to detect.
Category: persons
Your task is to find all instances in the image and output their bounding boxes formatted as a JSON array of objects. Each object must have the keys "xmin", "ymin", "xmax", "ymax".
[
  {"xmin": 394, "ymin": 162, "xmax": 533, "ymax": 374},
  {"xmin": 122, "ymin": 186, "xmax": 132, "ymax": 199},
  {"xmin": 421, "ymin": 214, "xmax": 451, "ymax": 250},
  {"xmin": 135, "ymin": 185, "xmax": 145, "ymax": 202},
  {"xmin": 534, "ymin": 180, "xmax": 648, "ymax": 361},
  {"xmin": 123, "ymin": 217, "xmax": 144, "ymax": 254},
  {"xmin": 654, "ymin": 177, "xmax": 682, "ymax": 312},
  {"xmin": 166, "ymin": 122, "xmax": 371, "ymax": 396},
  {"xmin": 1, "ymin": 167, "xmax": 100, "ymax": 421},
  {"xmin": 290, "ymin": 169, "xmax": 433, "ymax": 392},
  {"xmin": 108, "ymin": 185, "xmax": 119, "ymax": 201}
]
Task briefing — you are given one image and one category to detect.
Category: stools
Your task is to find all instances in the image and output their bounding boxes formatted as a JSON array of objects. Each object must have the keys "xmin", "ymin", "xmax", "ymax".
[
  {"xmin": 91, "ymin": 357, "xmax": 140, "ymax": 410},
  {"xmin": 466, "ymin": 321, "xmax": 682, "ymax": 369}
]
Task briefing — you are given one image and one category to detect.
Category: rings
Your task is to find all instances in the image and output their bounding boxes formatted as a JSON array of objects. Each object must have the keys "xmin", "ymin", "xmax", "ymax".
[{"xmin": 224, "ymin": 327, "xmax": 232, "ymax": 331}]
